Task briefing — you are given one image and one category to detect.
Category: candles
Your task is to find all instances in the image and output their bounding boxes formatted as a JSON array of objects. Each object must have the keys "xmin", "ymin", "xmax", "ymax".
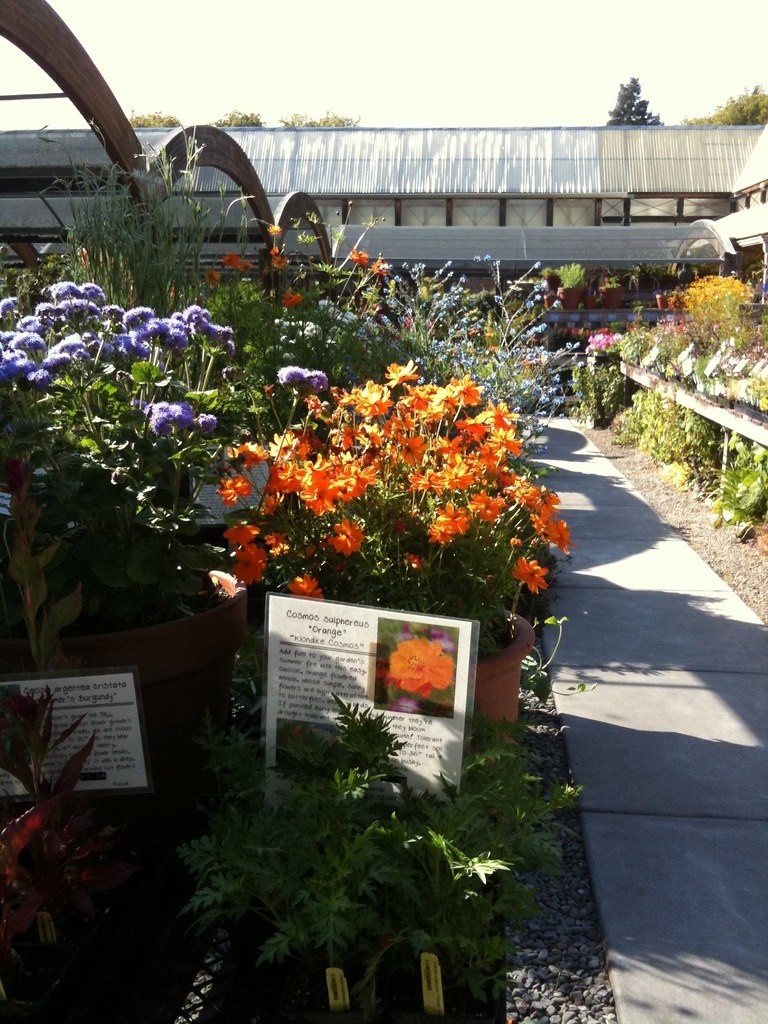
[{"xmin": 653, "ymin": 289, "xmax": 673, "ymax": 309}]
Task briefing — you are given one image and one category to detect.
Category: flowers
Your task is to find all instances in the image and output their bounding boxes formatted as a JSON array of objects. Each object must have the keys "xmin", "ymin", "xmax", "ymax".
[
  {"xmin": 80, "ymin": 192, "xmax": 767, "ymax": 460},
  {"xmin": 0, "ymin": 279, "xmax": 332, "ymax": 618},
  {"xmin": 210, "ymin": 357, "xmax": 575, "ymax": 664}
]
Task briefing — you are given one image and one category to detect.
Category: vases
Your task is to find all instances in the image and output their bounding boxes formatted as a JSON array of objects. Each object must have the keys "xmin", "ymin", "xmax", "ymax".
[
  {"xmin": 56, "ymin": 584, "xmax": 258, "ymax": 779},
  {"xmin": 469, "ymin": 604, "xmax": 540, "ymax": 767}
]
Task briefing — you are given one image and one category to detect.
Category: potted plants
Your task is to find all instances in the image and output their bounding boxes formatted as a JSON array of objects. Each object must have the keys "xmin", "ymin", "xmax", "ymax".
[
  {"xmin": 554, "ymin": 261, "xmax": 588, "ymax": 310},
  {"xmin": 621, "ymin": 260, "xmax": 660, "ymax": 292},
  {"xmin": 598, "ymin": 262, "xmax": 625, "ymax": 310},
  {"xmin": 543, "ymin": 290, "xmax": 558, "ymax": 311},
  {"xmin": 583, "ymin": 284, "xmax": 597, "ymax": 310},
  {"xmin": 538, "ymin": 266, "xmax": 560, "ymax": 293},
  {"xmin": 0, "ymin": 680, "xmax": 154, "ymax": 1024},
  {"xmin": 188, "ymin": 696, "xmax": 591, "ymax": 1024}
]
